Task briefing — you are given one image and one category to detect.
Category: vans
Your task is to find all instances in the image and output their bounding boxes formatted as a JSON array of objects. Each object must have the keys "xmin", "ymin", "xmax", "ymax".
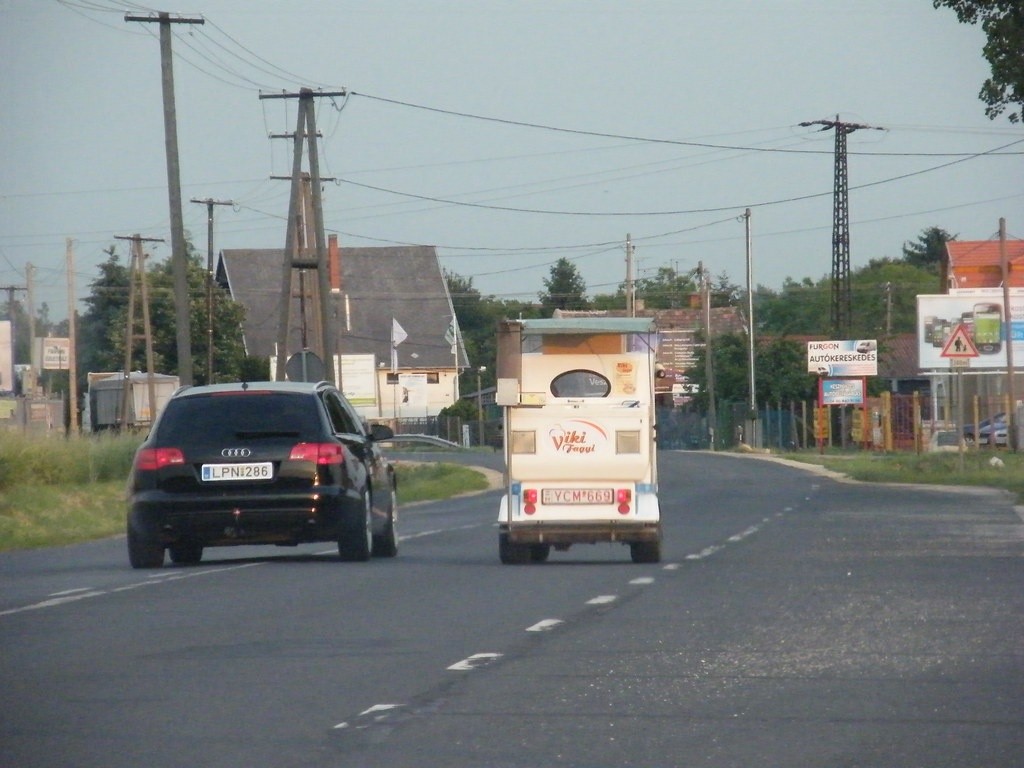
[{"xmin": 495, "ymin": 316, "xmax": 668, "ymax": 565}]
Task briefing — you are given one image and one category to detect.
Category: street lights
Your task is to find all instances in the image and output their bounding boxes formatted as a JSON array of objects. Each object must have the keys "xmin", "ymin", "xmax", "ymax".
[
  {"xmin": 885, "ymin": 281, "xmax": 893, "ymax": 335},
  {"xmin": 477, "ymin": 365, "xmax": 487, "ymax": 449}
]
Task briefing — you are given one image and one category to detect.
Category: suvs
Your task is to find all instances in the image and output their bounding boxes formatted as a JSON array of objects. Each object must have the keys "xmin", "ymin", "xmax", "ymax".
[{"xmin": 125, "ymin": 382, "xmax": 401, "ymax": 570}]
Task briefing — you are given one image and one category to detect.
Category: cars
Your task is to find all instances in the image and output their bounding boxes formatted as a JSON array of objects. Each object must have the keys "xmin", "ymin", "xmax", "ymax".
[{"xmin": 962, "ymin": 412, "xmax": 1009, "ymax": 447}]
